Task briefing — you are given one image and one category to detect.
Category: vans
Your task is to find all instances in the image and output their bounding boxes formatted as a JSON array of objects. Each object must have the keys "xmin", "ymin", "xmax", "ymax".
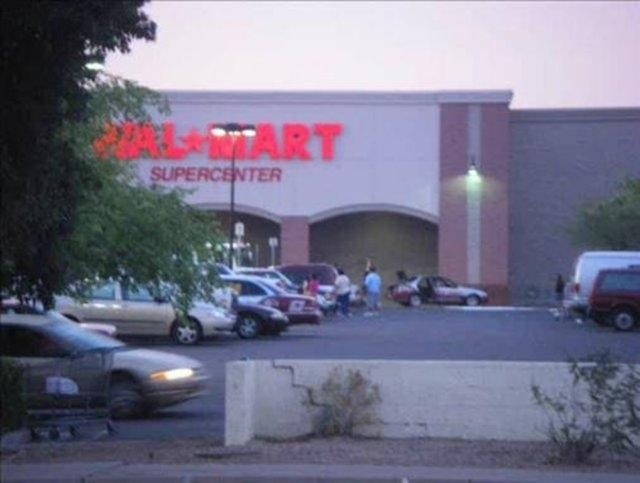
[{"xmin": 562, "ymin": 250, "xmax": 640, "ymax": 323}]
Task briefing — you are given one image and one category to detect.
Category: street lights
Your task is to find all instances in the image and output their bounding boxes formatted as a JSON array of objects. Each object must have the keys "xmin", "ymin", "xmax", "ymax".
[{"xmin": 210, "ymin": 121, "xmax": 258, "ymax": 268}]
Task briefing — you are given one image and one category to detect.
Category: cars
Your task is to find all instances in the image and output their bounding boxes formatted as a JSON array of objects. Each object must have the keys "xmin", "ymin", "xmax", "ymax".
[
  {"xmin": 586, "ymin": 265, "xmax": 640, "ymax": 331},
  {"xmin": 0, "ymin": 295, "xmax": 121, "ymax": 341},
  {"xmin": 0, "ymin": 313, "xmax": 208, "ymax": 420},
  {"xmin": 391, "ymin": 274, "xmax": 488, "ymax": 308}
]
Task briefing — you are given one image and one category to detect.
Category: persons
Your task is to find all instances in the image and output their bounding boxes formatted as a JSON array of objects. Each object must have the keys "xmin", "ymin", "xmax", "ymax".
[
  {"xmin": 362, "ymin": 268, "xmax": 383, "ymax": 317},
  {"xmin": 555, "ymin": 275, "xmax": 565, "ymax": 303},
  {"xmin": 334, "ymin": 269, "xmax": 353, "ymax": 319},
  {"xmin": 297, "ymin": 273, "xmax": 320, "ymax": 294}
]
